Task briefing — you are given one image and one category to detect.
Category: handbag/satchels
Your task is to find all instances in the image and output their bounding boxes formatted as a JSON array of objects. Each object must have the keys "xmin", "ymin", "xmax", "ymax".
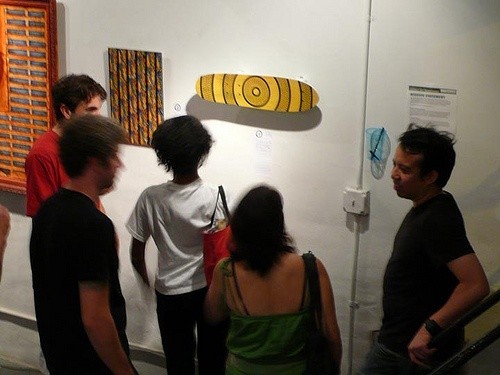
[
  {"xmin": 201, "ymin": 186, "xmax": 235, "ymax": 291},
  {"xmin": 300, "ymin": 252, "xmax": 340, "ymax": 375}
]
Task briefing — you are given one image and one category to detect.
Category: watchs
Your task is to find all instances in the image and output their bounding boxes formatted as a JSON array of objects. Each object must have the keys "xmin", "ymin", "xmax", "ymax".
[{"xmin": 425, "ymin": 319, "xmax": 442, "ymax": 335}]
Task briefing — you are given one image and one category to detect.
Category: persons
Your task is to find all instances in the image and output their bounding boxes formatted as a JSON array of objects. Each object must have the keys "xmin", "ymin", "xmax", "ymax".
[
  {"xmin": 205, "ymin": 184, "xmax": 343, "ymax": 375},
  {"xmin": 0, "ymin": 204, "xmax": 12, "ymax": 280},
  {"xmin": 24, "ymin": 72, "xmax": 108, "ymax": 217},
  {"xmin": 126, "ymin": 114, "xmax": 236, "ymax": 375},
  {"xmin": 29, "ymin": 114, "xmax": 141, "ymax": 375},
  {"xmin": 358, "ymin": 121, "xmax": 489, "ymax": 375}
]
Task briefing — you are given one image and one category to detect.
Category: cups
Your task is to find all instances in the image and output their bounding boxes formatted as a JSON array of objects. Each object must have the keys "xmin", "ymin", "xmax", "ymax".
[{"xmin": 366, "ymin": 127, "xmax": 385, "ymax": 161}]
